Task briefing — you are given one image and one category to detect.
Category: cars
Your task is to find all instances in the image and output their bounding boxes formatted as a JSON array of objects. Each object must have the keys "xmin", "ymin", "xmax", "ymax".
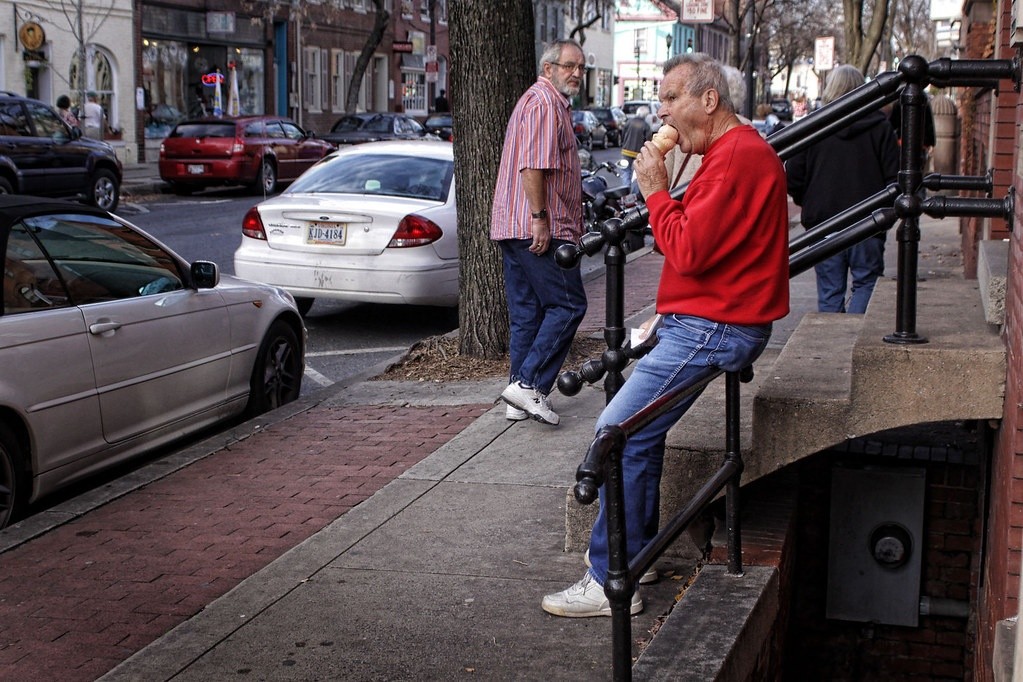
[
  {"xmin": 572, "ymin": 111, "xmax": 609, "ymax": 150},
  {"xmin": 423, "ymin": 113, "xmax": 453, "ymax": 142},
  {"xmin": 0, "ymin": 193, "xmax": 308, "ymax": 525},
  {"xmin": 157, "ymin": 115, "xmax": 339, "ymax": 195},
  {"xmin": 143, "ymin": 104, "xmax": 184, "ymax": 124},
  {"xmin": 771, "ymin": 100, "xmax": 792, "ymax": 122},
  {"xmin": 233, "ymin": 140, "xmax": 462, "ymax": 318}
]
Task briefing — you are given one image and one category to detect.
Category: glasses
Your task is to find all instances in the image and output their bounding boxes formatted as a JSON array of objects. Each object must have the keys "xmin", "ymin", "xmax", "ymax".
[{"xmin": 545, "ymin": 60, "xmax": 589, "ymax": 74}]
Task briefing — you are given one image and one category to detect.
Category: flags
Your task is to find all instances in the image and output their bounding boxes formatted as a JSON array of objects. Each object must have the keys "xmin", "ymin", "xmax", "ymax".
[
  {"xmin": 227, "ymin": 66, "xmax": 241, "ymax": 118},
  {"xmin": 213, "ymin": 73, "xmax": 222, "ymax": 120}
]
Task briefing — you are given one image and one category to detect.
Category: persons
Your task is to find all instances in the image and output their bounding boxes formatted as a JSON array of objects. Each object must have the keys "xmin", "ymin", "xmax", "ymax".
[
  {"xmin": 890, "ymin": 91, "xmax": 937, "ymax": 179},
  {"xmin": 55, "ymin": 94, "xmax": 79, "ymax": 127},
  {"xmin": 662, "ymin": 66, "xmax": 769, "ymax": 193},
  {"xmin": 757, "ymin": 102, "xmax": 786, "ymax": 138},
  {"xmin": 490, "ymin": 39, "xmax": 588, "ymax": 426},
  {"xmin": 620, "ymin": 106, "xmax": 653, "ymax": 200},
  {"xmin": 435, "ymin": 89, "xmax": 448, "ymax": 113},
  {"xmin": 541, "ymin": 54, "xmax": 790, "ymax": 616},
  {"xmin": 79, "ymin": 91, "xmax": 113, "ymax": 140},
  {"xmin": 790, "ymin": 86, "xmax": 810, "ymax": 123},
  {"xmin": 781, "ymin": 65, "xmax": 902, "ymax": 314}
]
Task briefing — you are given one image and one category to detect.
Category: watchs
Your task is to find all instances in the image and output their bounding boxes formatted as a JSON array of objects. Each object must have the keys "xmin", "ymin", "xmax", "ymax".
[{"xmin": 532, "ymin": 208, "xmax": 547, "ymax": 219}]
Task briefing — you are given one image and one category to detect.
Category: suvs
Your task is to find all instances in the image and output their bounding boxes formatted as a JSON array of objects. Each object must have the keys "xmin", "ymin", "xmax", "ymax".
[
  {"xmin": 622, "ymin": 101, "xmax": 663, "ymax": 132},
  {"xmin": 324, "ymin": 114, "xmax": 442, "ymax": 150},
  {"xmin": 0, "ymin": 91, "xmax": 123, "ymax": 213},
  {"xmin": 589, "ymin": 106, "xmax": 629, "ymax": 146}
]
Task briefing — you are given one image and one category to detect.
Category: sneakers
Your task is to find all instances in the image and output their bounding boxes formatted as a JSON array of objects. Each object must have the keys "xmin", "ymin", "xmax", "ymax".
[
  {"xmin": 541, "ymin": 568, "xmax": 644, "ymax": 617},
  {"xmin": 583, "ymin": 548, "xmax": 658, "ymax": 584},
  {"xmin": 505, "ymin": 396, "xmax": 554, "ymax": 420},
  {"xmin": 500, "ymin": 374, "xmax": 560, "ymax": 426}
]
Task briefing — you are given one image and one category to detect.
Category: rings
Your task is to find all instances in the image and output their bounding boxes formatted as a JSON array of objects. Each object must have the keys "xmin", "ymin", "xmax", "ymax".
[
  {"xmin": 637, "ymin": 158, "xmax": 644, "ymax": 163},
  {"xmin": 538, "ymin": 244, "xmax": 542, "ymax": 247}
]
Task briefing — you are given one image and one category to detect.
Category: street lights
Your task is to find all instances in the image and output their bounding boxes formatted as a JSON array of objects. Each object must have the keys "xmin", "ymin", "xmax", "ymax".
[
  {"xmin": 290, "ymin": 0, "xmax": 307, "ymax": 128},
  {"xmin": 665, "ymin": 34, "xmax": 672, "ymax": 62}
]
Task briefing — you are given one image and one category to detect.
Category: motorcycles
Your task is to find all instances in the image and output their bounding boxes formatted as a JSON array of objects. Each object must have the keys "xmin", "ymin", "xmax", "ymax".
[{"xmin": 579, "ymin": 160, "xmax": 646, "ymax": 253}]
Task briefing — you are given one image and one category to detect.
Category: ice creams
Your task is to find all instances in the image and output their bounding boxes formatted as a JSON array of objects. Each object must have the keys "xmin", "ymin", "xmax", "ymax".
[{"xmin": 651, "ymin": 124, "xmax": 678, "ymax": 155}]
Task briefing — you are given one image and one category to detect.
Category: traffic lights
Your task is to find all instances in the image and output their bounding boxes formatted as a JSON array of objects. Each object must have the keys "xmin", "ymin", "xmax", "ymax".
[
  {"xmin": 642, "ymin": 78, "xmax": 646, "ymax": 86},
  {"xmin": 687, "ymin": 39, "xmax": 692, "ymax": 52}
]
